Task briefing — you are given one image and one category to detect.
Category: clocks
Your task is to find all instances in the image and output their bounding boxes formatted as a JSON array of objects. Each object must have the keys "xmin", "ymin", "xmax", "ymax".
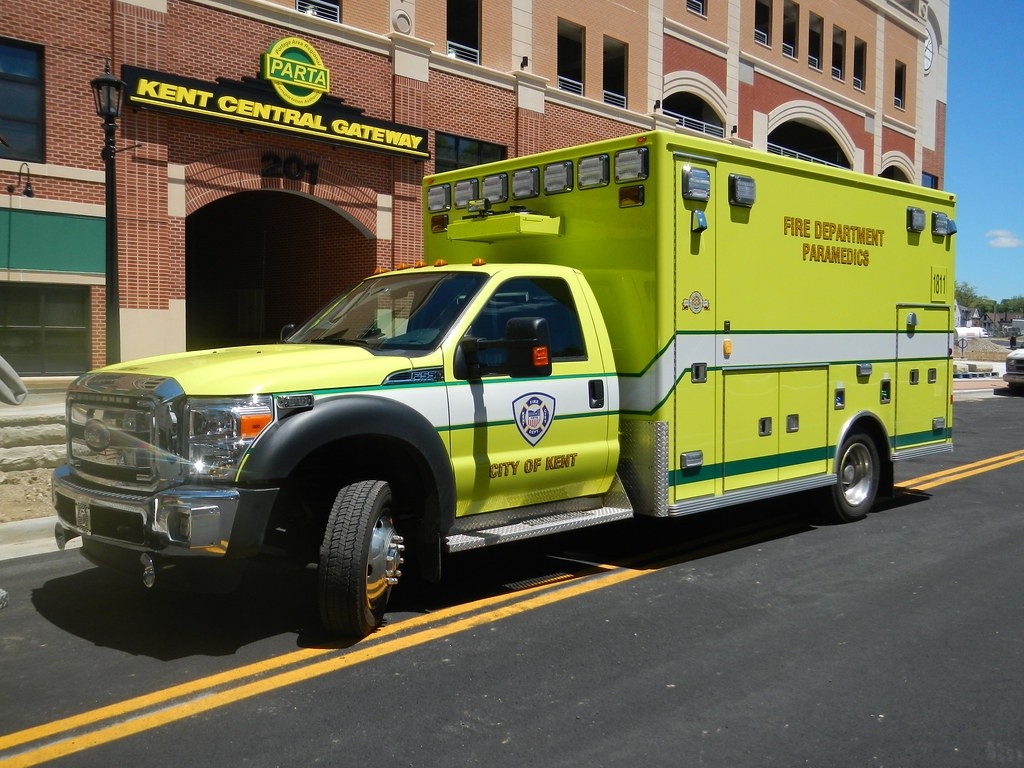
[{"xmin": 924, "ymin": 28, "xmax": 934, "ymax": 75}]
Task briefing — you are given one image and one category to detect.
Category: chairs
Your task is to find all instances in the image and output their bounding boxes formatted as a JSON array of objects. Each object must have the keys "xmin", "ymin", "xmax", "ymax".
[{"xmin": 498, "ymin": 306, "xmax": 561, "ymax": 356}]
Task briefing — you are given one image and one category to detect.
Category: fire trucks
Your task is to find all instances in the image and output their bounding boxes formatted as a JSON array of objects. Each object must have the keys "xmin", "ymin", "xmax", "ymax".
[{"xmin": 50, "ymin": 129, "xmax": 957, "ymax": 638}]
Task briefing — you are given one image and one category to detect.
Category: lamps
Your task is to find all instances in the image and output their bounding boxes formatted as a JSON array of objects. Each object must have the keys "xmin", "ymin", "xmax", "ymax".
[{"xmin": 7, "ymin": 163, "xmax": 34, "ymax": 198}]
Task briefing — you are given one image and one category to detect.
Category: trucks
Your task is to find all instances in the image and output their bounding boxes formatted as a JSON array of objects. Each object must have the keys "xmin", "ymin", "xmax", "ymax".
[{"xmin": 956, "ymin": 327, "xmax": 988, "ymax": 338}]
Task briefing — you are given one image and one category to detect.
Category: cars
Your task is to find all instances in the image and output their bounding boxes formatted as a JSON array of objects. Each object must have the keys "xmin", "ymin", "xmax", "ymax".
[{"xmin": 1003, "ymin": 341, "xmax": 1024, "ymax": 392}]
